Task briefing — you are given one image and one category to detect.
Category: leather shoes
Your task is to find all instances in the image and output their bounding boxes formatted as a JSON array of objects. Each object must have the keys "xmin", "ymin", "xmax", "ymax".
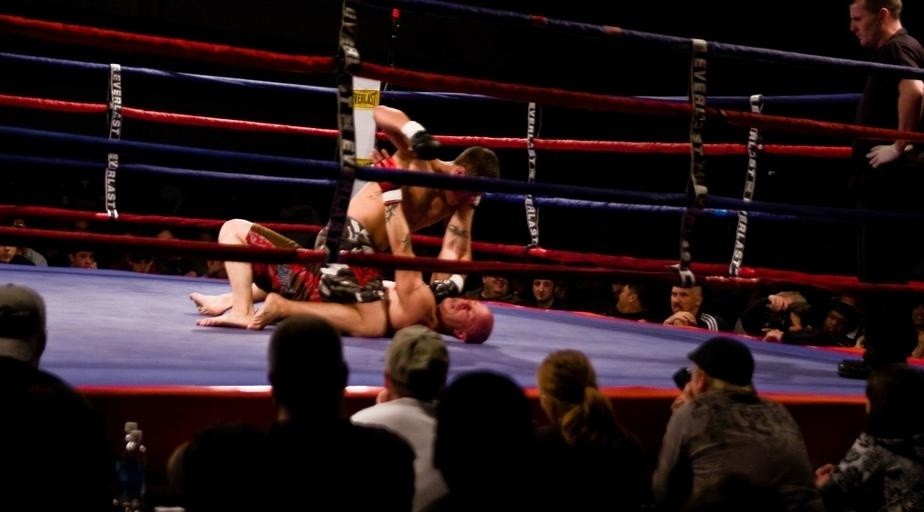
[{"xmin": 836, "ymin": 342, "xmax": 909, "ymax": 380}]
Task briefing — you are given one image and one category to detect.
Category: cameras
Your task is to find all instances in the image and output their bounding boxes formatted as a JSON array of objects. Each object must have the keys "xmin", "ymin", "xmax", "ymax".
[
  {"xmin": 673, "ymin": 367, "xmax": 692, "ymax": 391},
  {"xmin": 763, "ymin": 308, "xmax": 790, "ymax": 332}
]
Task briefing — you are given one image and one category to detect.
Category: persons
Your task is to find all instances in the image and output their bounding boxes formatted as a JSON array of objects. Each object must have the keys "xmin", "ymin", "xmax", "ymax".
[
  {"xmin": 167, "ymin": 311, "xmax": 419, "ymax": 512},
  {"xmin": 909, "ymin": 307, "xmax": 923, "ymax": 358},
  {"xmin": 537, "ymin": 348, "xmax": 641, "ymax": 510},
  {"xmin": 190, "ymin": 146, "xmax": 494, "ymax": 344},
  {"xmin": 248, "ymin": 100, "xmax": 503, "ymax": 339},
  {"xmin": 350, "ymin": 324, "xmax": 447, "ymax": 512},
  {"xmin": 1, "ymin": 284, "xmax": 113, "ymax": 512},
  {"xmin": 430, "ymin": 368, "xmax": 595, "ymax": 511},
  {"xmin": 813, "ymin": 360, "xmax": 922, "ymax": 512},
  {"xmin": 647, "ymin": 337, "xmax": 814, "ymax": 512},
  {"xmin": 835, "ymin": 0, "xmax": 924, "ymax": 385},
  {"xmin": 472, "ymin": 270, "xmax": 866, "ymax": 349},
  {"xmin": 1, "ymin": 218, "xmax": 227, "ymax": 278}
]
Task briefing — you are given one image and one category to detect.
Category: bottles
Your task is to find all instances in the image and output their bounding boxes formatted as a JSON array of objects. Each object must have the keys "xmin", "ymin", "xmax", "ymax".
[{"xmin": 121, "ymin": 421, "xmax": 149, "ymax": 505}]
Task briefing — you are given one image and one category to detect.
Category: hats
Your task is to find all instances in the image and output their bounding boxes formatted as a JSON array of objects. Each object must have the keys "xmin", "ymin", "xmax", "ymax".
[
  {"xmin": 384, "ymin": 323, "xmax": 450, "ymax": 390},
  {"xmin": 685, "ymin": 335, "xmax": 757, "ymax": 388}
]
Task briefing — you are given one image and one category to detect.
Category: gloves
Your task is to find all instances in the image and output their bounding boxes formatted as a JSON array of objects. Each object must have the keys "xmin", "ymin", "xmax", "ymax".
[
  {"xmin": 401, "ymin": 120, "xmax": 440, "ymax": 161},
  {"xmin": 430, "ymin": 274, "xmax": 465, "ymax": 304},
  {"xmin": 372, "ymin": 156, "xmax": 403, "ymax": 204},
  {"xmin": 865, "ymin": 143, "xmax": 914, "ymax": 169},
  {"xmin": 318, "ymin": 272, "xmax": 386, "ymax": 302}
]
